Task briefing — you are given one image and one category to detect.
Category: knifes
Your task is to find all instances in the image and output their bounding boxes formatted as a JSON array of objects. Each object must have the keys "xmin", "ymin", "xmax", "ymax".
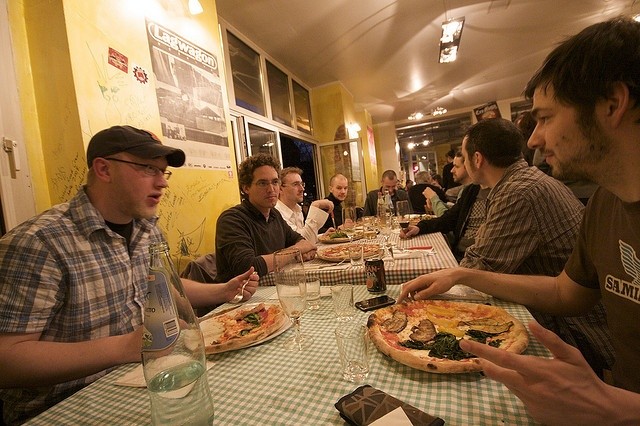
[
  {"xmin": 330, "ymin": 208, "xmax": 336, "ymax": 230},
  {"xmin": 394, "ymin": 246, "xmax": 432, "ymax": 249}
]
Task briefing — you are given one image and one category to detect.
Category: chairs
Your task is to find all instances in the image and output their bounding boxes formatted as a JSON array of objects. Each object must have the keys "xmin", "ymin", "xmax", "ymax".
[{"xmin": 191, "ymin": 252, "xmax": 218, "ymax": 315}]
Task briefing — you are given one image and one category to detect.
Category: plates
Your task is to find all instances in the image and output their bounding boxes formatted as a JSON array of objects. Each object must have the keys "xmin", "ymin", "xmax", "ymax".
[{"xmin": 182, "ymin": 303, "xmax": 296, "ymax": 351}]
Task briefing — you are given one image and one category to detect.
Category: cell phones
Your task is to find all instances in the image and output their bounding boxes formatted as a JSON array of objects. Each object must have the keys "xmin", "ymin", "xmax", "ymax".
[{"xmin": 355, "ymin": 295, "xmax": 396, "ymax": 313}]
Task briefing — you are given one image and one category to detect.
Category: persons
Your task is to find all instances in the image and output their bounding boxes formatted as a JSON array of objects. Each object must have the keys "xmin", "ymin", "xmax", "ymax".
[
  {"xmin": 400, "ymin": 181, "xmax": 492, "ymax": 264},
  {"xmin": 317, "ymin": 172, "xmax": 349, "ymax": 234},
  {"xmin": 215, "ymin": 152, "xmax": 317, "ymax": 285},
  {"xmin": 459, "ymin": 118, "xmax": 617, "ymax": 379},
  {"xmin": 0, "ymin": 126, "xmax": 260, "ymax": 425},
  {"xmin": 429, "ymin": 167, "xmax": 441, "ymax": 184},
  {"xmin": 513, "ymin": 110, "xmax": 538, "ymax": 163},
  {"xmin": 395, "ymin": 177, "xmax": 404, "ymax": 190},
  {"xmin": 402, "ymin": 178, "xmax": 413, "ymax": 191},
  {"xmin": 421, "ymin": 151, "xmax": 471, "ymax": 247},
  {"xmin": 362, "ymin": 170, "xmax": 414, "ymax": 216},
  {"xmin": 398, "ymin": 14, "xmax": 640, "ymax": 426},
  {"xmin": 408, "ymin": 171, "xmax": 450, "ymax": 214},
  {"xmin": 274, "ymin": 167, "xmax": 334, "ymax": 242},
  {"xmin": 442, "ymin": 149, "xmax": 462, "ymax": 192}
]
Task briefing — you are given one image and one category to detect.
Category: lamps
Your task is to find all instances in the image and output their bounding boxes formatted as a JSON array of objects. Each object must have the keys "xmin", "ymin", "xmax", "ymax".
[{"xmin": 439, "ymin": 0, "xmax": 465, "ymax": 64}]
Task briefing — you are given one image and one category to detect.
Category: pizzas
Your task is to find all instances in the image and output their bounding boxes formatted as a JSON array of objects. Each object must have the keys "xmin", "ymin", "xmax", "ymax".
[
  {"xmin": 368, "ymin": 300, "xmax": 530, "ymax": 375},
  {"xmin": 192, "ymin": 303, "xmax": 285, "ymax": 356},
  {"xmin": 319, "ymin": 231, "xmax": 362, "ymax": 243},
  {"xmin": 407, "ymin": 214, "xmax": 434, "ymax": 223}
]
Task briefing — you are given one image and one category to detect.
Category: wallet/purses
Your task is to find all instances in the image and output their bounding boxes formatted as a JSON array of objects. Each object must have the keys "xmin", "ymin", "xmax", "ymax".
[{"xmin": 335, "ymin": 384, "xmax": 446, "ymax": 426}]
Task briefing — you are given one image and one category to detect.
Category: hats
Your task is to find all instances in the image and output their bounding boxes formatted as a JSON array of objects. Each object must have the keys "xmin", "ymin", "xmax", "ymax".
[{"xmin": 87, "ymin": 124, "xmax": 186, "ymax": 169}]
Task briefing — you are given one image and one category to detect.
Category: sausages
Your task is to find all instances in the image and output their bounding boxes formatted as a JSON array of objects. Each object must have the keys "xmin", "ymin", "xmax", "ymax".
[
  {"xmin": 241, "ymin": 304, "xmax": 264, "ymax": 317},
  {"xmin": 383, "ymin": 310, "xmax": 407, "ymax": 332},
  {"xmin": 409, "ymin": 319, "xmax": 435, "ymax": 342}
]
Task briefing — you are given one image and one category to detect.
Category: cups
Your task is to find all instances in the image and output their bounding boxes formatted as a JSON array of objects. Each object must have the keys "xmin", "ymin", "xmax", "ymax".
[
  {"xmin": 362, "ymin": 216, "xmax": 376, "ymax": 240},
  {"xmin": 298, "ymin": 276, "xmax": 320, "ymax": 311},
  {"xmin": 349, "ymin": 246, "xmax": 364, "ymax": 266},
  {"xmin": 380, "ymin": 246, "xmax": 395, "ymax": 267},
  {"xmin": 330, "ymin": 283, "xmax": 355, "ymax": 322},
  {"xmin": 336, "ymin": 322, "xmax": 370, "ymax": 383}
]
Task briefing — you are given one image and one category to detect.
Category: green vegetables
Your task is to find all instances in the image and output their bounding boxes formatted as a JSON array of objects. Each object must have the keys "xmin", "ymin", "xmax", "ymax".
[
  {"xmin": 396, "ymin": 339, "xmax": 434, "ymax": 349},
  {"xmin": 244, "ymin": 313, "xmax": 261, "ymax": 326},
  {"xmin": 429, "ymin": 333, "xmax": 477, "ymax": 360},
  {"xmin": 464, "ymin": 330, "xmax": 496, "ymax": 347},
  {"xmin": 329, "ymin": 232, "xmax": 350, "ymax": 240},
  {"xmin": 240, "ymin": 326, "xmax": 256, "ymax": 336}
]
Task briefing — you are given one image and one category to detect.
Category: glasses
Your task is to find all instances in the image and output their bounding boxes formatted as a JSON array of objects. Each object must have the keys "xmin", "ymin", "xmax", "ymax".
[
  {"xmin": 104, "ymin": 156, "xmax": 172, "ymax": 180},
  {"xmin": 291, "ymin": 182, "xmax": 307, "ymax": 188},
  {"xmin": 252, "ymin": 179, "xmax": 283, "ymax": 188}
]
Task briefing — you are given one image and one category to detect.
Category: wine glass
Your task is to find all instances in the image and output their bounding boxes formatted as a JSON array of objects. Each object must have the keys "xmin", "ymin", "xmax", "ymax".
[
  {"xmin": 396, "ymin": 200, "xmax": 412, "ymax": 241},
  {"xmin": 345, "ymin": 206, "xmax": 357, "ymax": 230},
  {"xmin": 343, "ymin": 207, "xmax": 356, "ymax": 243},
  {"xmin": 273, "ymin": 248, "xmax": 314, "ymax": 351}
]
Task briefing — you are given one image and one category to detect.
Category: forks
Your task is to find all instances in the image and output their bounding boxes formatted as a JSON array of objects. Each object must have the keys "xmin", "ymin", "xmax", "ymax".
[{"xmin": 229, "ymin": 272, "xmax": 255, "ymax": 303}]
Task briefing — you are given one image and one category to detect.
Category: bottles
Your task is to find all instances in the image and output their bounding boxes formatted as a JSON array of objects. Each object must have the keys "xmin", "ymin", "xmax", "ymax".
[
  {"xmin": 385, "ymin": 191, "xmax": 396, "ymax": 222},
  {"xmin": 377, "ymin": 192, "xmax": 384, "ymax": 217},
  {"xmin": 141, "ymin": 242, "xmax": 215, "ymax": 425}
]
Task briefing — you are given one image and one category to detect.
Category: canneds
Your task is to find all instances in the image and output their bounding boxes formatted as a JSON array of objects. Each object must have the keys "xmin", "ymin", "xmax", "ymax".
[{"xmin": 365, "ymin": 257, "xmax": 386, "ymax": 295}]
